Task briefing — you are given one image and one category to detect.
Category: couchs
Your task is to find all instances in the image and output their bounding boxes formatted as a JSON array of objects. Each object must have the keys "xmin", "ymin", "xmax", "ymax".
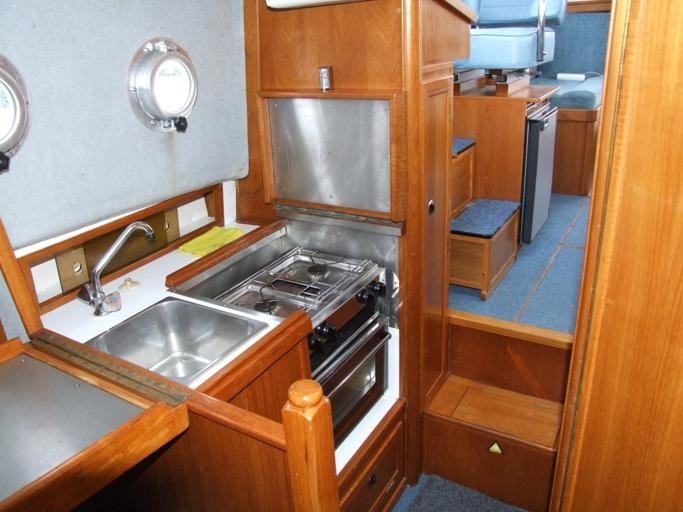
[{"xmin": 530, "ymin": 12, "xmax": 610, "ymax": 197}]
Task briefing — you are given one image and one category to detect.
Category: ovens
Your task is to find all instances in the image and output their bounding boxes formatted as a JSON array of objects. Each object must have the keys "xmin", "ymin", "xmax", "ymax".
[{"xmin": 311, "ymin": 312, "xmax": 392, "ymax": 452}]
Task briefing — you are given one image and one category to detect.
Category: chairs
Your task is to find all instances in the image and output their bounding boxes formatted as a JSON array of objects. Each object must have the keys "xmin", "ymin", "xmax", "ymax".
[{"xmin": 452, "ymin": 0, "xmax": 567, "ymax": 70}]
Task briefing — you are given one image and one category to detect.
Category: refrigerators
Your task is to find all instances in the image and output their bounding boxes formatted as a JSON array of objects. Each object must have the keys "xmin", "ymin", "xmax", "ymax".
[{"xmin": 520, "ymin": 105, "xmax": 558, "ymax": 246}]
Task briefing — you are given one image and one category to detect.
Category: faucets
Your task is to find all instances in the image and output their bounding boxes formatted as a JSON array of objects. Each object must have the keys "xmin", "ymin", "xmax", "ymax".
[{"xmin": 79, "ymin": 218, "xmax": 158, "ymax": 317}]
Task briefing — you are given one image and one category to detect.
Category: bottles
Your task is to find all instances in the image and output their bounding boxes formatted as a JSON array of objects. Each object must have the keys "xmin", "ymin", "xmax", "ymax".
[{"xmin": 319, "ymin": 65, "xmax": 330, "ymax": 92}]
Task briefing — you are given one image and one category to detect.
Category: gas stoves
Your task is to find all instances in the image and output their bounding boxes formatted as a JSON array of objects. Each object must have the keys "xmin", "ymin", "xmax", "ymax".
[{"xmin": 213, "ymin": 245, "xmax": 386, "ymax": 370}]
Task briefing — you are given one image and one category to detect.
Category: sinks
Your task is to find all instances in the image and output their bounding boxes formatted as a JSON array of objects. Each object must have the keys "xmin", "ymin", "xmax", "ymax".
[{"xmin": 84, "ymin": 295, "xmax": 261, "ymax": 383}]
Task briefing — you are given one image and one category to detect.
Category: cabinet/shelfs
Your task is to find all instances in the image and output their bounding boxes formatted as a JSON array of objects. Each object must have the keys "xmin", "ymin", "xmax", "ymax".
[
  {"xmin": 335, "ymin": 399, "xmax": 406, "ymax": 512},
  {"xmin": 401, "ymin": 75, "xmax": 453, "ymax": 412}
]
